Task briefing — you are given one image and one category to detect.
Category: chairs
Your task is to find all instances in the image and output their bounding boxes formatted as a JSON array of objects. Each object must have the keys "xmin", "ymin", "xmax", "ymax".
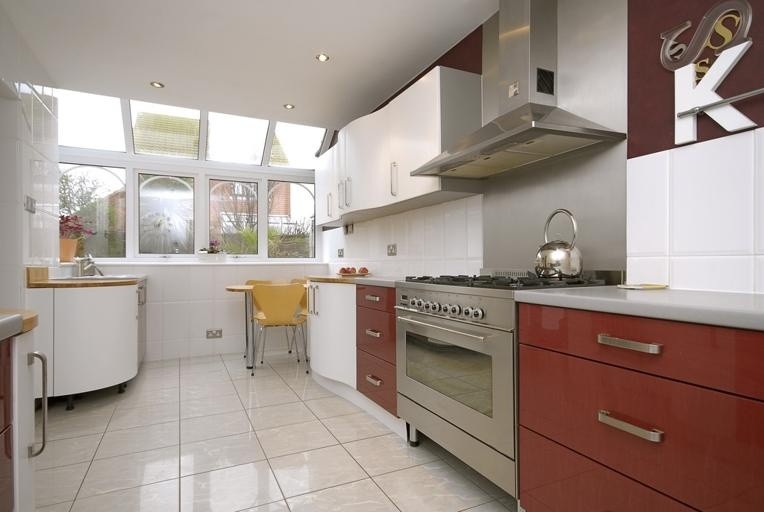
[{"xmin": 242, "ymin": 279, "xmax": 308, "ymax": 376}]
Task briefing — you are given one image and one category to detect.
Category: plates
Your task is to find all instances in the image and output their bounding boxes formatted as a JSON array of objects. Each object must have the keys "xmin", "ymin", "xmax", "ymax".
[{"xmin": 336, "ymin": 272, "xmax": 372, "ymax": 276}]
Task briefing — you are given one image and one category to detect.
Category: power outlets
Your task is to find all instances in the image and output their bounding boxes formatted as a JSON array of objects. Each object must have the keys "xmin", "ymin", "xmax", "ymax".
[
  {"xmin": 24, "ymin": 196, "xmax": 36, "ymax": 213},
  {"xmin": 206, "ymin": 329, "xmax": 222, "ymax": 339}
]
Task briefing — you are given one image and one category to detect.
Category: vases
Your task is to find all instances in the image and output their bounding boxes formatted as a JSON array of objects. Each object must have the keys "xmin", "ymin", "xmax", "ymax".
[
  {"xmin": 205, "ymin": 253, "xmax": 217, "ymax": 263},
  {"xmin": 59, "ymin": 239, "xmax": 78, "ymax": 262}
]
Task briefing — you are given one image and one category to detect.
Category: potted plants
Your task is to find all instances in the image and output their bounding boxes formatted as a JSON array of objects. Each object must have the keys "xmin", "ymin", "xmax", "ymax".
[{"xmin": 198, "ymin": 247, "xmax": 207, "ymax": 263}]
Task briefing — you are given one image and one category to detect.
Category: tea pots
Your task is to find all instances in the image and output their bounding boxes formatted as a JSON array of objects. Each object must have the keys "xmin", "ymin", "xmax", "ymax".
[{"xmin": 535, "ymin": 208, "xmax": 585, "ymax": 279}]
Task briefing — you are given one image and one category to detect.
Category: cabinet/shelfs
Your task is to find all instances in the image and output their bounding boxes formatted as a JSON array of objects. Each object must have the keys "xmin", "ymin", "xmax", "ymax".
[
  {"xmin": 0, "ymin": 276, "xmax": 149, "ymax": 512},
  {"xmin": 307, "ymin": 282, "xmax": 357, "ymax": 390},
  {"xmin": 314, "ymin": 65, "xmax": 482, "ymax": 232},
  {"xmin": 515, "ymin": 304, "xmax": 764, "ymax": 512},
  {"xmin": 356, "ymin": 285, "xmax": 396, "ymax": 416}
]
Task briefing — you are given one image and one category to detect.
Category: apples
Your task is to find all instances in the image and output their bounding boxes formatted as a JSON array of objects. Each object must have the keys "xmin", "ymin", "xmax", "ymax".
[{"xmin": 340, "ymin": 267, "xmax": 368, "ymax": 274}]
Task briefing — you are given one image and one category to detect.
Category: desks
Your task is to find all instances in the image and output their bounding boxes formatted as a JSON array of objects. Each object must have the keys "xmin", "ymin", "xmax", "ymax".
[{"xmin": 225, "ymin": 284, "xmax": 309, "ymax": 369}]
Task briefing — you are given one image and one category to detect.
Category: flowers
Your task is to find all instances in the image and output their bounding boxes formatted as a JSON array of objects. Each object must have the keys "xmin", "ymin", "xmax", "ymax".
[
  {"xmin": 58, "ymin": 214, "xmax": 97, "ymax": 247},
  {"xmin": 206, "ymin": 239, "xmax": 222, "ymax": 252}
]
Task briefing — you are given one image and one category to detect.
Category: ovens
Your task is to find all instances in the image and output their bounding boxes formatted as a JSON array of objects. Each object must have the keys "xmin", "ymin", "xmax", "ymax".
[{"xmin": 395, "ymin": 286, "xmax": 517, "ymax": 461}]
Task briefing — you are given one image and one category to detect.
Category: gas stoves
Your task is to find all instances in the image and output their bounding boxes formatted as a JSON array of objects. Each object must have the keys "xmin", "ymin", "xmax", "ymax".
[{"xmin": 404, "ymin": 272, "xmax": 606, "ymax": 291}]
